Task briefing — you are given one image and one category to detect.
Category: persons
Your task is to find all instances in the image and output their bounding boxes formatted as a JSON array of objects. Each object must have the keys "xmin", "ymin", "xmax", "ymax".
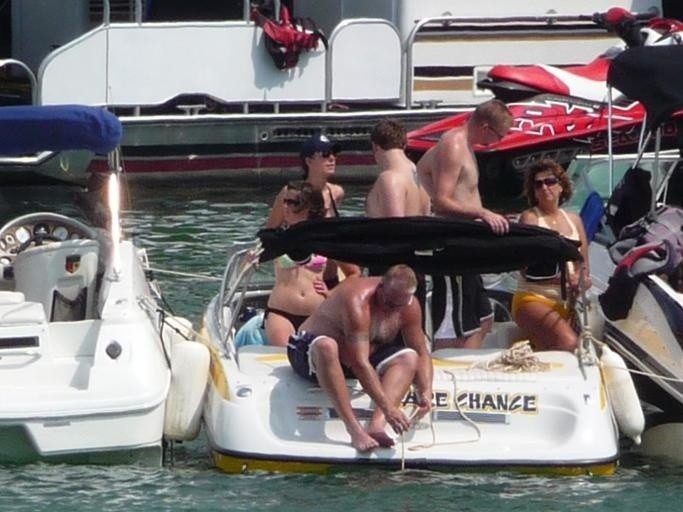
[
  {"xmin": 365, "ymin": 120, "xmax": 434, "ymax": 324},
  {"xmin": 417, "ymin": 100, "xmax": 514, "ymax": 351},
  {"xmin": 264, "ymin": 180, "xmax": 361, "ymax": 348},
  {"xmin": 287, "ymin": 265, "xmax": 433, "ymax": 451},
  {"xmin": 265, "ymin": 134, "xmax": 344, "ymax": 290},
  {"xmin": 510, "ymin": 159, "xmax": 592, "ymax": 354}
]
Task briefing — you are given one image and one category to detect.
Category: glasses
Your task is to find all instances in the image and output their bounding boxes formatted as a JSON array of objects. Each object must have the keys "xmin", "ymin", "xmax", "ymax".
[
  {"xmin": 531, "ymin": 177, "xmax": 559, "ymax": 190},
  {"xmin": 312, "ymin": 149, "xmax": 337, "ymax": 158},
  {"xmin": 480, "ymin": 123, "xmax": 505, "ymax": 143}
]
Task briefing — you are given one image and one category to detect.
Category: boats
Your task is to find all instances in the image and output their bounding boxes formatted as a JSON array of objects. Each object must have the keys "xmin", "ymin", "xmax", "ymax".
[
  {"xmin": 1, "ymin": 210, "xmax": 210, "ymax": 476},
  {"xmin": 0, "ymin": 1, "xmax": 683, "ymax": 189},
  {"xmin": 199, "ymin": 199, "xmax": 683, "ymax": 480}
]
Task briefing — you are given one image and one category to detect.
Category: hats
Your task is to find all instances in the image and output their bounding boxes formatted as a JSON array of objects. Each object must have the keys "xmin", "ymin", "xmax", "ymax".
[{"xmin": 301, "ymin": 133, "xmax": 341, "ymax": 154}]
